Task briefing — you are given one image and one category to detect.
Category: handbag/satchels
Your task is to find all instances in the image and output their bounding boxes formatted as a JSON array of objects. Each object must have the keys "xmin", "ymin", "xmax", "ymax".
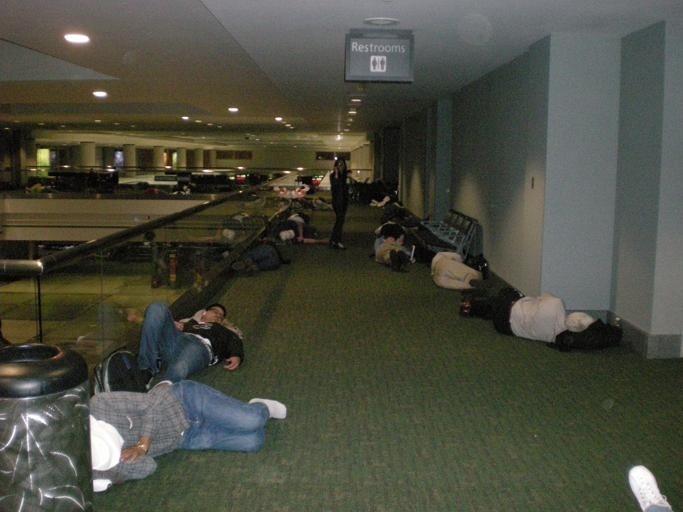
[
  {"xmin": 556, "ymin": 319, "xmax": 623, "ymax": 350},
  {"xmin": 94, "ymin": 347, "xmax": 146, "ymax": 394},
  {"xmin": 463, "ymin": 254, "xmax": 489, "ymax": 276}
]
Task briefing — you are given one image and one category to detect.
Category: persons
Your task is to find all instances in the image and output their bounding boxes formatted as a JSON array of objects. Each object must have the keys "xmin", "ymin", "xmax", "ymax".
[
  {"xmin": 231, "ymin": 195, "xmax": 328, "ymax": 276},
  {"xmin": 330, "ymin": 159, "xmax": 349, "ymax": 249},
  {"xmin": 135, "ymin": 302, "xmax": 245, "ymax": 390},
  {"xmin": 90, "ymin": 379, "xmax": 287, "ymax": 484},
  {"xmin": 370, "ymin": 197, "xmax": 591, "ymax": 342}
]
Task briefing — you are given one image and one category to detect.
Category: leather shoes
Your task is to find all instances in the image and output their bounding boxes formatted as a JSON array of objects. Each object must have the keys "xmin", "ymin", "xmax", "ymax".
[
  {"xmin": 328, "ymin": 239, "xmax": 345, "ymax": 249},
  {"xmin": 462, "ymin": 298, "xmax": 470, "ymax": 316}
]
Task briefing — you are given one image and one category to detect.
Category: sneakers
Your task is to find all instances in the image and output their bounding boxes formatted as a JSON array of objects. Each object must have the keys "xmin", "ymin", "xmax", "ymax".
[
  {"xmin": 389, "ymin": 249, "xmax": 411, "ymax": 272},
  {"xmin": 249, "ymin": 398, "xmax": 287, "ymax": 419},
  {"xmin": 142, "ymin": 368, "xmax": 153, "ymax": 389},
  {"xmin": 232, "ymin": 258, "xmax": 258, "ymax": 277},
  {"xmin": 147, "ymin": 380, "xmax": 173, "ymax": 394}
]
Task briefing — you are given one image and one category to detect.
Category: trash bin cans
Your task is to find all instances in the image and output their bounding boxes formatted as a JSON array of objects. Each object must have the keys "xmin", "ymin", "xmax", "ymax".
[{"xmin": 1, "ymin": 340, "xmax": 95, "ymax": 512}]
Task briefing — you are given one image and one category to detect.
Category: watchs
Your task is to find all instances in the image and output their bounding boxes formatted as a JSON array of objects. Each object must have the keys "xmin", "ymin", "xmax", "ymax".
[{"xmin": 137, "ymin": 443, "xmax": 149, "ymax": 452}]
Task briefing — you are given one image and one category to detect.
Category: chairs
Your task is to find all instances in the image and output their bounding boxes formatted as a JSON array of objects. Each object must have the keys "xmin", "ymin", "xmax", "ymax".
[{"xmin": 411, "ymin": 210, "xmax": 478, "ymax": 263}]
